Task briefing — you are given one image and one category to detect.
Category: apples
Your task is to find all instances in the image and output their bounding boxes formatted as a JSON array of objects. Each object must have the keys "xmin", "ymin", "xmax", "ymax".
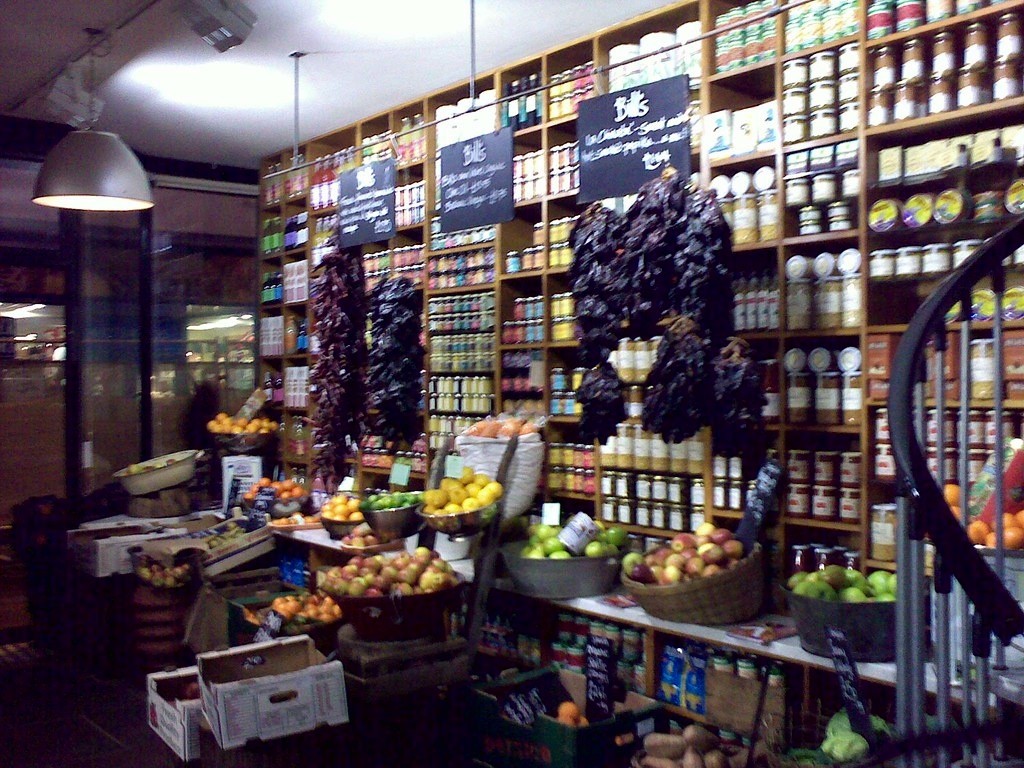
[
  {"xmin": 245, "ymin": 467, "xmax": 503, "ymax": 597},
  {"xmin": 520, "ymin": 521, "xmax": 744, "ymax": 587},
  {"xmin": 136, "ymin": 555, "xmax": 191, "ymax": 588},
  {"xmin": 787, "ymin": 565, "xmax": 899, "ymax": 603}
]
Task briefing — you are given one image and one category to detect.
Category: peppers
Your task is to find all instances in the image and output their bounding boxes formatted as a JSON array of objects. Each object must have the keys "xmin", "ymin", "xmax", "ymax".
[{"xmin": 307, "ymin": 221, "xmax": 372, "ymax": 491}]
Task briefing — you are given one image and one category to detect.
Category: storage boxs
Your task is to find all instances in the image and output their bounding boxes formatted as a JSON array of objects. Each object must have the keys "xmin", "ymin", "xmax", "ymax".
[
  {"xmin": 64, "ymin": 523, "xmax": 190, "ymax": 578},
  {"xmin": 196, "ymin": 634, "xmax": 349, "ymax": 751},
  {"xmin": 184, "ymin": 512, "xmax": 275, "ymax": 579},
  {"xmin": 145, "ymin": 665, "xmax": 213, "ymax": 764},
  {"xmin": 468, "ymin": 664, "xmax": 662, "ymax": 768}
]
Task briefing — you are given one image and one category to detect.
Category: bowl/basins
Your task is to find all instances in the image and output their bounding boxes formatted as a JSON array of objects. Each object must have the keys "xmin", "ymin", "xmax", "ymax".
[
  {"xmin": 321, "ymin": 517, "xmax": 365, "ymax": 536},
  {"xmin": 320, "ymin": 571, "xmax": 465, "ymax": 640},
  {"xmin": 502, "ymin": 541, "xmax": 628, "ymax": 598},
  {"xmin": 112, "ymin": 449, "xmax": 198, "ymax": 495},
  {"xmin": 244, "ymin": 498, "xmax": 313, "ymax": 520},
  {"xmin": 359, "ymin": 493, "xmax": 420, "ymax": 539},
  {"xmin": 415, "ymin": 503, "xmax": 499, "ymax": 534},
  {"xmin": 779, "ymin": 581, "xmax": 928, "ymax": 663},
  {"xmin": 208, "ymin": 431, "xmax": 272, "ymax": 453}
]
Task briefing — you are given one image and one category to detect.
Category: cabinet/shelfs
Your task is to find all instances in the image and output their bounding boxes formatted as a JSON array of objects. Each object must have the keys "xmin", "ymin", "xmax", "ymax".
[
  {"xmin": 252, "ymin": 0, "xmax": 1024, "ymax": 604},
  {"xmin": 484, "ymin": 579, "xmax": 1000, "ymax": 768}
]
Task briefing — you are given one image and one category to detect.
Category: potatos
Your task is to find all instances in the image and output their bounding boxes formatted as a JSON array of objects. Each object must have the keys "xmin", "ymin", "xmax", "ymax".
[{"xmin": 635, "ymin": 724, "xmax": 732, "ymax": 768}]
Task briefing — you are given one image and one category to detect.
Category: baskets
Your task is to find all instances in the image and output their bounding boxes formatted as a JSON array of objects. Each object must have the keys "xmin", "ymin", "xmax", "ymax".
[
  {"xmin": 631, "ymin": 730, "xmax": 782, "ymax": 768},
  {"xmin": 620, "ymin": 534, "xmax": 767, "ymax": 625}
]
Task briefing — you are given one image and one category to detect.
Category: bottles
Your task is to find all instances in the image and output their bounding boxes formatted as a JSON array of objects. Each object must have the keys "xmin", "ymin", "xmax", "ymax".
[
  {"xmin": 288, "ymin": 416, "xmax": 308, "ymax": 459},
  {"xmin": 261, "ymin": 272, "xmax": 282, "ymax": 305},
  {"xmin": 732, "ymin": 272, "xmax": 746, "ymax": 331},
  {"xmin": 397, "ymin": 114, "xmax": 426, "ymax": 167},
  {"xmin": 262, "ymin": 372, "xmax": 272, "ymax": 405},
  {"xmin": 769, "ymin": 272, "xmax": 779, "ymax": 332},
  {"xmin": 285, "ymin": 212, "xmax": 308, "ymax": 250},
  {"xmin": 758, "ymin": 271, "xmax": 769, "ymax": 331},
  {"xmin": 260, "ymin": 217, "xmax": 280, "ymax": 255},
  {"xmin": 286, "ymin": 315, "xmax": 308, "ymax": 354},
  {"xmin": 310, "ymin": 146, "xmax": 355, "ymax": 209},
  {"xmin": 745, "ymin": 271, "xmax": 757, "ymax": 331},
  {"xmin": 312, "ymin": 215, "xmax": 338, "ymax": 265},
  {"xmin": 274, "ymin": 374, "xmax": 283, "ymax": 405}
]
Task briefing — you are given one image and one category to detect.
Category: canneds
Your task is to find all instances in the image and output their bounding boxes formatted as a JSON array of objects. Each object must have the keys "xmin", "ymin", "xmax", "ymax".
[
  {"xmin": 359, "ymin": 1, "xmax": 1024, "ymax": 571},
  {"xmin": 553, "ymin": 611, "xmax": 645, "ymax": 696}
]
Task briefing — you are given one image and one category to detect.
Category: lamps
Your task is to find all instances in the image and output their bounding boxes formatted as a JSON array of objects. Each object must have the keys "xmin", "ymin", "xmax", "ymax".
[{"xmin": 30, "ymin": 28, "xmax": 157, "ymax": 212}]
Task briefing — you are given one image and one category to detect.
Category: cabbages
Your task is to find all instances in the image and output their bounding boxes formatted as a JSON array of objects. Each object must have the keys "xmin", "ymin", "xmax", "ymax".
[{"xmin": 782, "ymin": 707, "xmax": 891, "ymax": 768}]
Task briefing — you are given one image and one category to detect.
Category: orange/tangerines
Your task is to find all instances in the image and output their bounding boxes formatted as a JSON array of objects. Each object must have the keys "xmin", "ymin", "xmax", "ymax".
[
  {"xmin": 206, "ymin": 412, "xmax": 278, "ymax": 435},
  {"xmin": 272, "ymin": 593, "xmax": 342, "ymax": 629}
]
